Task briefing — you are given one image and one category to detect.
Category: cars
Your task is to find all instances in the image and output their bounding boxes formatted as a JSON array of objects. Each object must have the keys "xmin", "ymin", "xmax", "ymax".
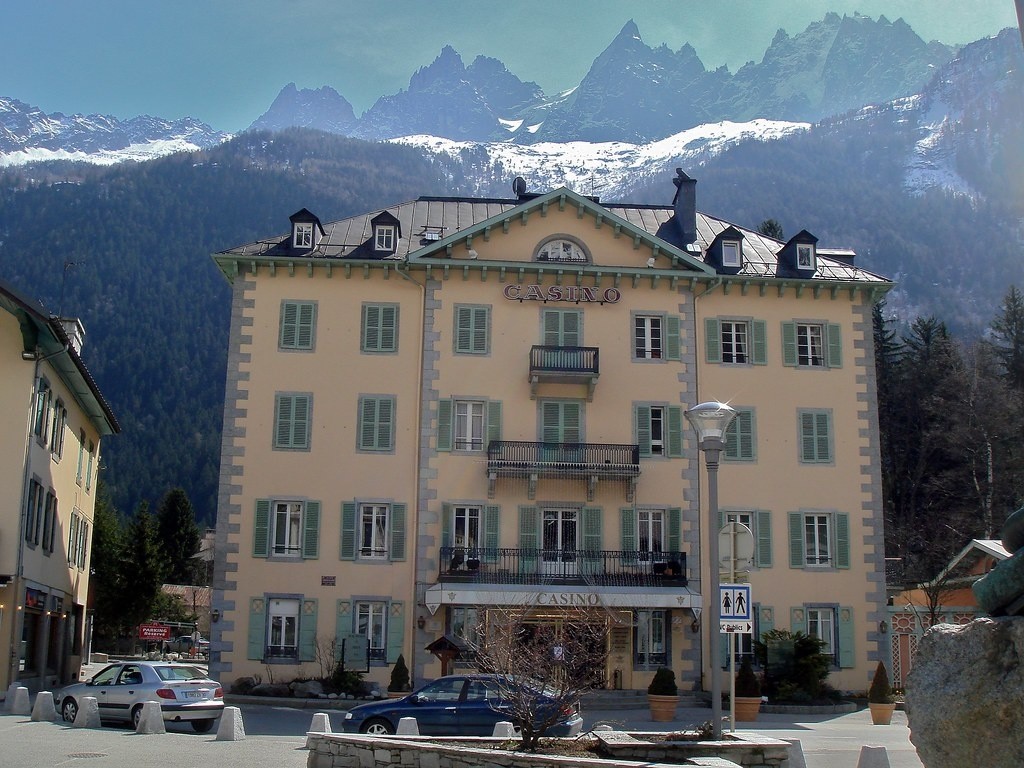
[
  {"xmin": 166, "ymin": 635, "xmax": 210, "ymax": 654},
  {"xmin": 342, "ymin": 674, "xmax": 584, "ymax": 737},
  {"xmin": 55, "ymin": 661, "xmax": 225, "ymax": 733}
]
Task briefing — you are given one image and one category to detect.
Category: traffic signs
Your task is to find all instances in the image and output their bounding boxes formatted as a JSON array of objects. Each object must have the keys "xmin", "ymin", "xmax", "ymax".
[{"xmin": 718, "ymin": 583, "xmax": 753, "ymax": 634}]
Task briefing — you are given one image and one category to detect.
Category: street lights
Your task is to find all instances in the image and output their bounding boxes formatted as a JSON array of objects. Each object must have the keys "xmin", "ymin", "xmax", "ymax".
[{"xmin": 685, "ymin": 401, "xmax": 738, "ymax": 738}]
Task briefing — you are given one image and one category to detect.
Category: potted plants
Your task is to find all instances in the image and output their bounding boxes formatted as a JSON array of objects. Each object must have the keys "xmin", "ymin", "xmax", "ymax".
[
  {"xmin": 386, "ymin": 653, "xmax": 414, "ymax": 699},
  {"xmin": 867, "ymin": 660, "xmax": 896, "ymax": 724},
  {"xmin": 647, "ymin": 666, "xmax": 681, "ymax": 722},
  {"xmin": 731, "ymin": 658, "xmax": 762, "ymax": 722}
]
